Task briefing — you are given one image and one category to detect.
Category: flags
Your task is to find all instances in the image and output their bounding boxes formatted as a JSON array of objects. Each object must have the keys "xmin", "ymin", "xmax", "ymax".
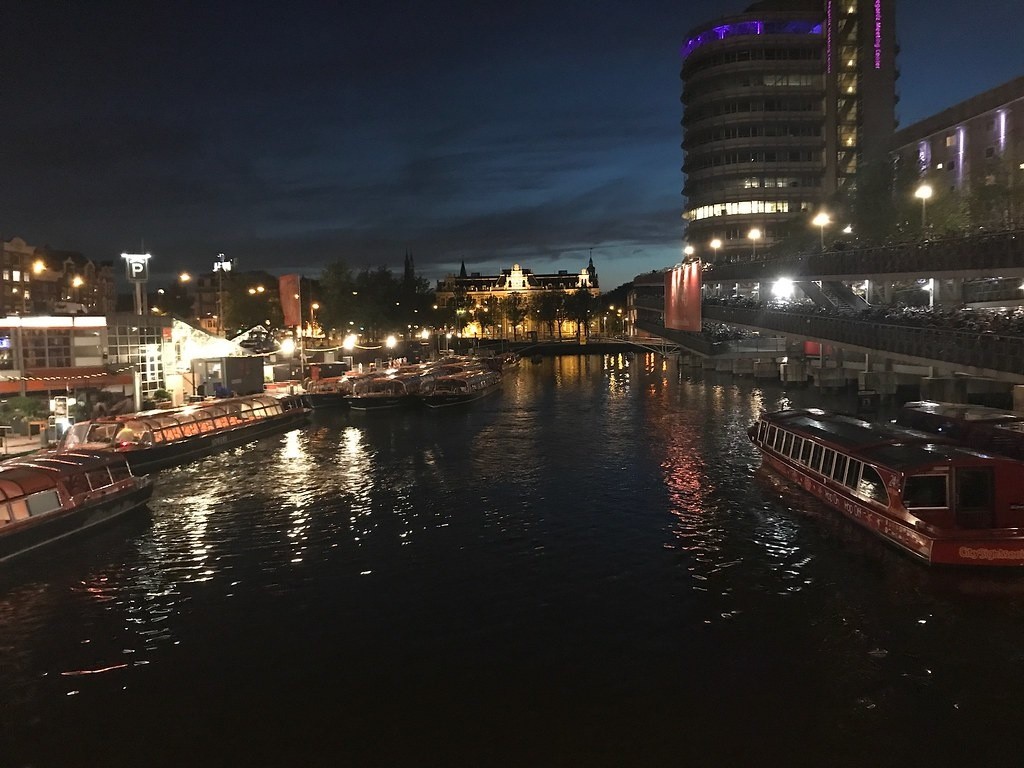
[{"xmin": 278, "ymin": 273, "xmax": 300, "ymax": 326}]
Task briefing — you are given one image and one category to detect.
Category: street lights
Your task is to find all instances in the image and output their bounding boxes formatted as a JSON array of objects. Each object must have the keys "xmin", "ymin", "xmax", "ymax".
[
  {"xmin": 213, "ymin": 254, "xmax": 231, "ymax": 331},
  {"xmin": 712, "ymin": 240, "xmax": 719, "ymax": 259},
  {"xmin": 919, "ymin": 184, "xmax": 931, "ymax": 226},
  {"xmin": 750, "ymin": 229, "xmax": 758, "ymax": 253},
  {"xmin": 817, "ymin": 212, "xmax": 827, "ymax": 247}
]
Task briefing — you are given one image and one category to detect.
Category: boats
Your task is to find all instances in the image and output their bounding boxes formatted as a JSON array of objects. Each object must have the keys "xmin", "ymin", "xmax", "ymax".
[
  {"xmin": 297, "ymin": 370, "xmax": 391, "ymax": 408},
  {"xmin": 0, "ymin": 448, "xmax": 155, "ymax": 565},
  {"xmin": 747, "ymin": 408, "xmax": 1024, "ymax": 569},
  {"xmin": 343, "ymin": 369, "xmax": 448, "ymax": 411},
  {"xmin": 56, "ymin": 393, "xmax": 316, "ymax": 473},
  {"xmin": 434, "ymin": 355, "xmax": 521, "ymax": 373},
  {"xmin": 414, "ymin": 369, "xmax": 503, "ymax": 409},
  {"xmin": 888, "ymin": 400, "xmax": 1023, "ymax": 462}
]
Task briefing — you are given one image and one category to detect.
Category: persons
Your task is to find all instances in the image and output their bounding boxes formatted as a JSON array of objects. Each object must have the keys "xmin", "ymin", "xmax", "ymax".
[{"xmin": 196, "ymin": 381, "xmax": 207, "ymax": 400}]
